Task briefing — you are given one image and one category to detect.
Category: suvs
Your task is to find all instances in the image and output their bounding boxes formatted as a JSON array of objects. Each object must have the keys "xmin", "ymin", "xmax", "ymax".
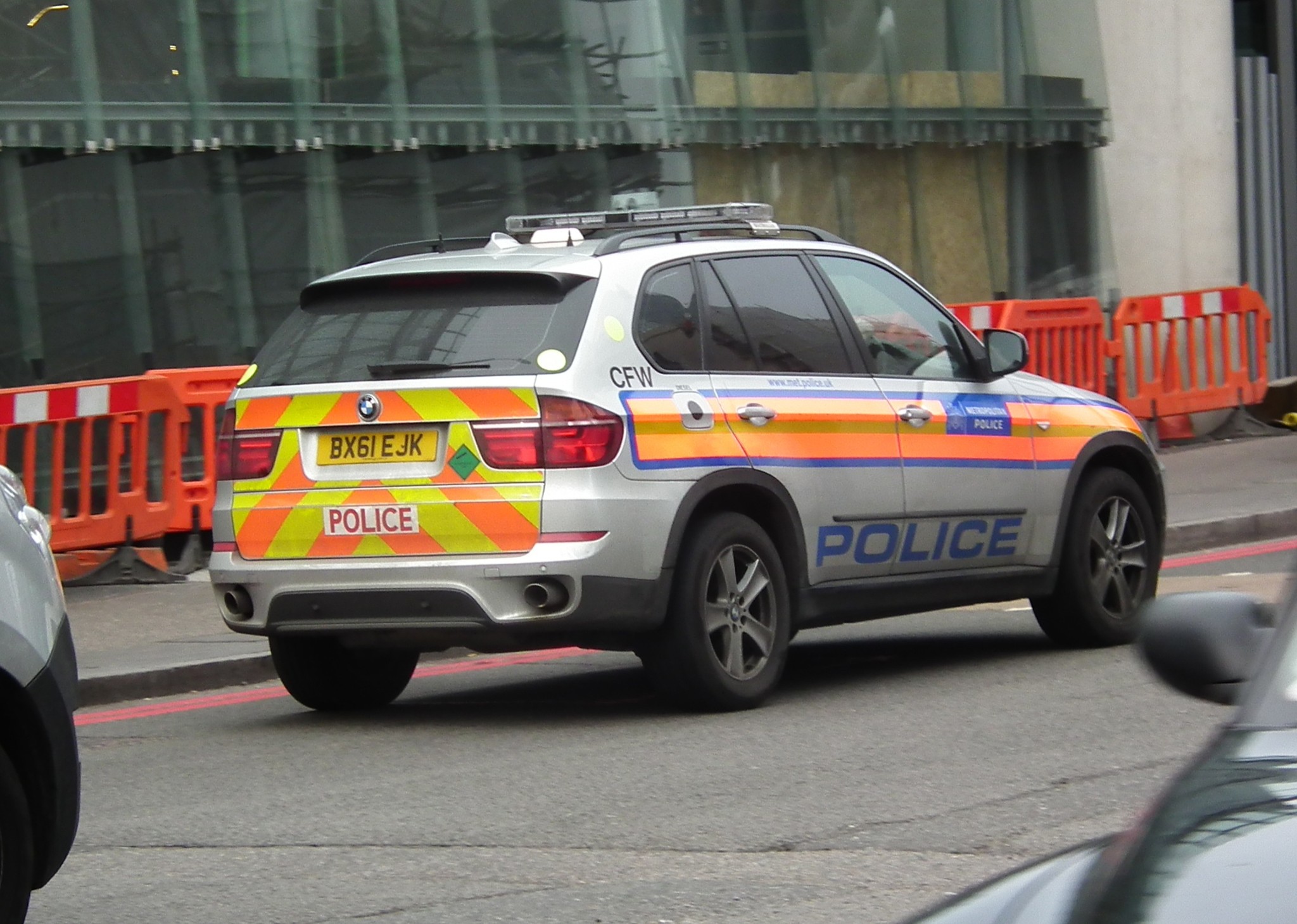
[{"xmin": 205, "ymin": 205, "xmax": 1168, "ymax": 717}]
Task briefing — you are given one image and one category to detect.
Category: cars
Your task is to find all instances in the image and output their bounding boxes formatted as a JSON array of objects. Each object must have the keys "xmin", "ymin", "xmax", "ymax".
[
  {"xmin": 0, "ymin": 466, "xmax": 83, "ymax": 924},
  {"xmin": 888, "ymin": 539, "xmax": 1297, "ymax": 923}
]
